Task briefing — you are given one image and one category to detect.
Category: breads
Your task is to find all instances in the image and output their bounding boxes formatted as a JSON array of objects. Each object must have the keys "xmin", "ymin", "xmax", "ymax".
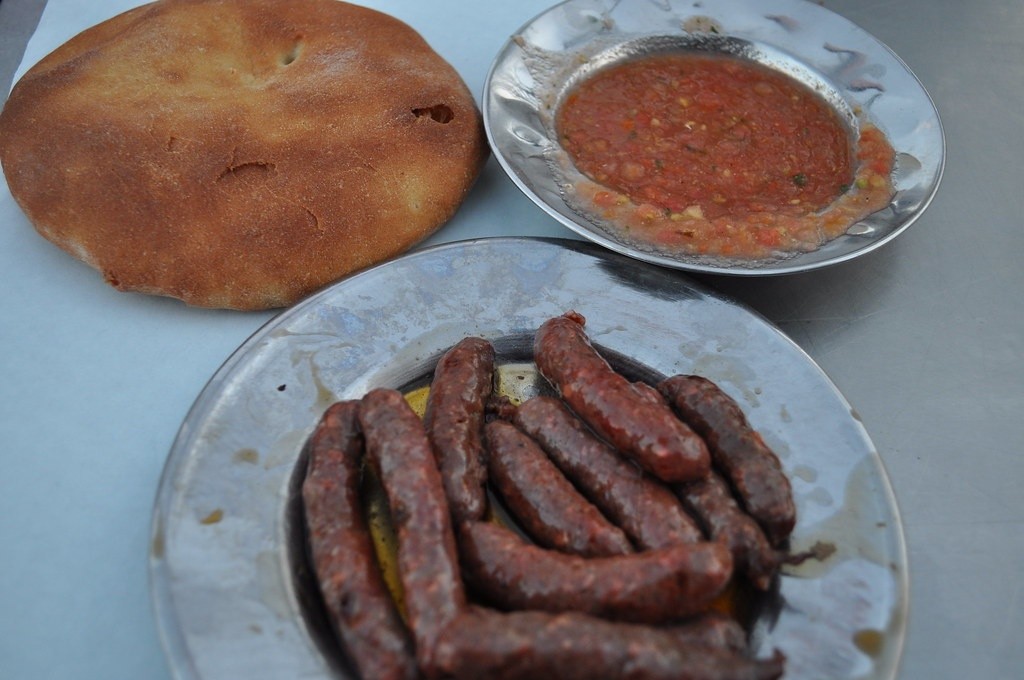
[{"xmin": 0, "ymin": 2, "xmax": 486, "ymax": 314}]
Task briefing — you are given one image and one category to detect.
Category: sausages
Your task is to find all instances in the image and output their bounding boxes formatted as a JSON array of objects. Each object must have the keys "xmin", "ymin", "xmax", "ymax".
[{"xmin": 298, "ymin": 315, "xmax": 797, "ymax": 680}]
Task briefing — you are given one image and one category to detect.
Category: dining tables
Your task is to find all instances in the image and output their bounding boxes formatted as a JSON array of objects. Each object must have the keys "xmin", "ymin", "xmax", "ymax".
[{"xmin": 1, "ymin": 2, "xmax": 1024, "ymax": 680}]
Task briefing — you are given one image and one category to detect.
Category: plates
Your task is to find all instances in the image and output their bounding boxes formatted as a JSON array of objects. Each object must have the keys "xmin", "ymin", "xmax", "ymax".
[
  {"xmin": 151, "ymin": 238, "xmax": 911, "ymax": 679},
  {"xmin": 483, "ymin": 0, "xmax": 949, "ymax": 275}
]
